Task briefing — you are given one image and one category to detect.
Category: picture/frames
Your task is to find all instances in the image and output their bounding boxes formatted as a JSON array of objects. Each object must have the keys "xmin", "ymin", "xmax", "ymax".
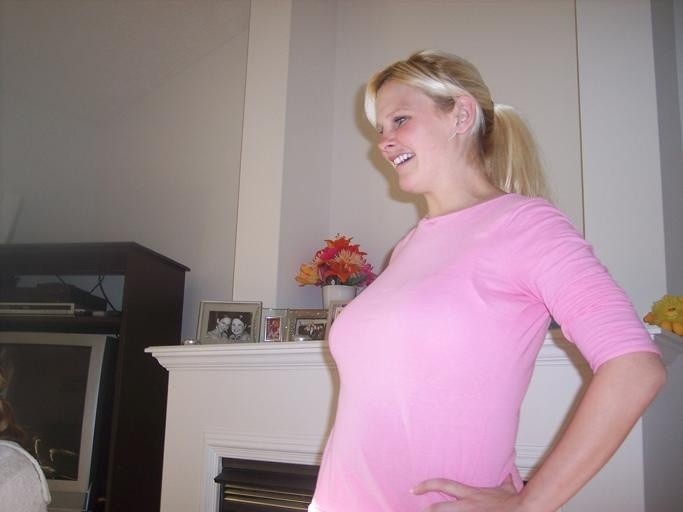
[{"xmin": 196, "ymin": 300, "xmax": 353, "ymax": 344}]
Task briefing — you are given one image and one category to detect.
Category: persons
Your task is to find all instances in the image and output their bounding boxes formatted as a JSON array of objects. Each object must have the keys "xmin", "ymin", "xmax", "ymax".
[
  {"xmin": 206, "ymin": 314, "xmax": 250, "ymax": 341},
  {"xmin": 268, "ymin": 321, "xmax": 279, "ymax": 338},
  {"xmin": 305, "ymin": 48, "xmax": 667, "ymax": 512}
]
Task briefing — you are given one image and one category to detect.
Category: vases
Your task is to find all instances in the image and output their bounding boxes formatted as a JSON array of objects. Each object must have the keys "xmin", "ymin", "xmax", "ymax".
[{"xmin": 322, "ymin": 285, "xmax": 356, "ymax": 309}]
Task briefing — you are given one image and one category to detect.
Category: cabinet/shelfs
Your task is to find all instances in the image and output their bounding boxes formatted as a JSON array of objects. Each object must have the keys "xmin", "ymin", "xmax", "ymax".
[{"xmin": 0, "ymin": 241, "xmax": 191, "ymax": 512}]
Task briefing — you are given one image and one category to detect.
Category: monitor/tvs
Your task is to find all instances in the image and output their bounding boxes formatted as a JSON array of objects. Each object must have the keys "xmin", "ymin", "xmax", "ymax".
[{"xmin": 0, "ymin": 322, "xmax": 119, "ymax": 512}]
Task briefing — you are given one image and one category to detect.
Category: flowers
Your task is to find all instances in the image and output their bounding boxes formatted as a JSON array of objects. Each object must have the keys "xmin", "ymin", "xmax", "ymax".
[{"xmin": 294, "ymin": 232, "xmax": 377, "ymax": 287}]
